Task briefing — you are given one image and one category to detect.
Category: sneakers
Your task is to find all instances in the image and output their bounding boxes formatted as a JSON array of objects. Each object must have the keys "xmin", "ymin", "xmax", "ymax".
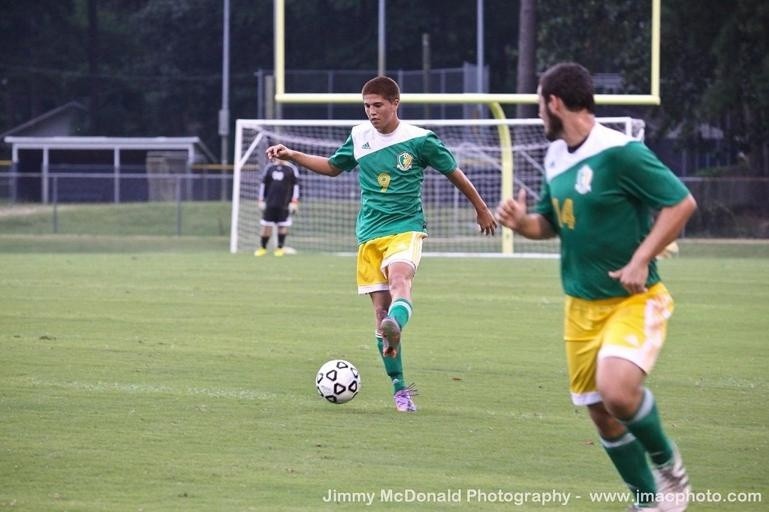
[
  {"xmin": 380, "ymin": 317, "xmax": 401, "ymax": 359},
  {"xmin": 254, "ymin": 247, "xmax": 285, "ymax": 258},
  {"xmin": 393, "ymin": 387, "xmax": 417, "ymax": 413},
  {"xmin": 651, "ymin": 438, "xmax": 691, "ymax": 512}
]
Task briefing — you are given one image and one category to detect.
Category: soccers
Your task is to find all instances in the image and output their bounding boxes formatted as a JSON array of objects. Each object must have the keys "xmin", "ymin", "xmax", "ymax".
[{"xmin": 315, "ymin": 360, "xmax": 361, "ymax": 403}]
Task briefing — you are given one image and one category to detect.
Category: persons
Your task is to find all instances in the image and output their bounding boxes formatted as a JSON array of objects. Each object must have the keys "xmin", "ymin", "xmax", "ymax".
[
  {"xmin": 252, "ymin": 157, "xmax": 301, "ymax": 258},
  {"xmin": 264, "ymin": 75, "xmax": 498, "ymax": 414},
  {"xmin": 491, "ymin": 57, "xmax": 701, "ymax": 512}
]
